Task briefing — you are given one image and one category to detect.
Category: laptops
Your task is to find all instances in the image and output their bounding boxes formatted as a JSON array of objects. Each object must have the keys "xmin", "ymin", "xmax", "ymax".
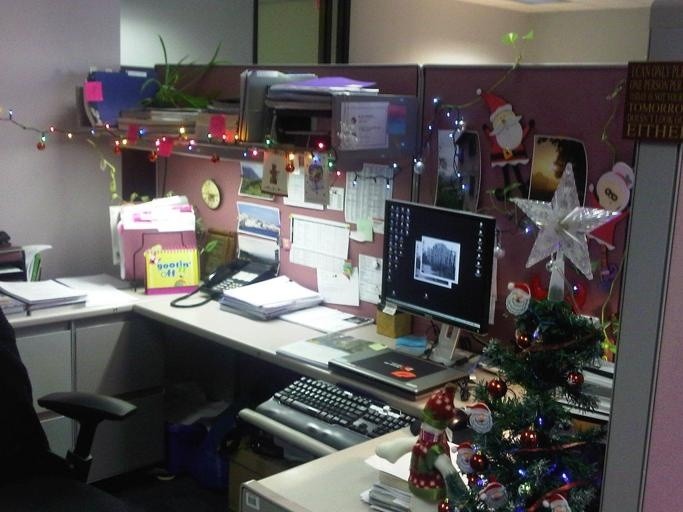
[{"xmin": 326, "ymin": 341, "xmax": 469, "ymax": 403}]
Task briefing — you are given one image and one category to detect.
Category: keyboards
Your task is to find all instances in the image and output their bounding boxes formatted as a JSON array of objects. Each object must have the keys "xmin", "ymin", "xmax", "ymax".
[{"xmin": 254, "ymin": 374, "xmax": 417, "ymax": 452}]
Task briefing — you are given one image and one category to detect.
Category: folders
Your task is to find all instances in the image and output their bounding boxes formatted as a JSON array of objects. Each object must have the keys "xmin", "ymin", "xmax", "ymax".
[{"xmin": 87, "ymin": 66, "xmax": 159, "ymax": 128}]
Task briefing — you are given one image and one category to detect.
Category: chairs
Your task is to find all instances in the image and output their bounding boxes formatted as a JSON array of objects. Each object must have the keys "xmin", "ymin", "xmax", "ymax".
[{"xmin": 0, "ymin": 310, "xmax": 145, "ymax": 512}]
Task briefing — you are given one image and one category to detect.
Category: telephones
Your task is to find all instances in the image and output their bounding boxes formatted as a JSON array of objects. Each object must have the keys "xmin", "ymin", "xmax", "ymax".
[{"xmin": 199, "ymin": 260, "xmax": 274, "ymax": 299}]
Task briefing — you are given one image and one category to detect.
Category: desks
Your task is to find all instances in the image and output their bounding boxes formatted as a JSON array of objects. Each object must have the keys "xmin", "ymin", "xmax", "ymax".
[{"xmin": 6, "ymin": 281, "xmax": 595, "ymax": 512}]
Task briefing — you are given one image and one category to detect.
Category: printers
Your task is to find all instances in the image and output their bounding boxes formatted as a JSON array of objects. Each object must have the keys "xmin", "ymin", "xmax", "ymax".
[{"xmin": 0, "ymin": 229, "xmax": 29, "ymax": 286}]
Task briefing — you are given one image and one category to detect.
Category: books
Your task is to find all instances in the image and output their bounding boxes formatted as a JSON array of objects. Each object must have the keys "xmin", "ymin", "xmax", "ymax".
[
  {"xmin": 0, "ymin": 276, "xmax": 87, "ymax": 321},
  {"xmin": 115, "ymin": 96, "xmax": 241, "ymax": 140},
  {"xmin": 463, "ymin": 343, "xmax": 615, "ymax": 427},
  {"xmin": 360, "ymin": 439, "xmax": 471, "ymax": 512},
  {"xmin": 238, "ymin": 69, "xmax": 379, "ymax": 143},
  {"xmin": 276, "ymin": 332, "xmax": 387, "ymax": 369}
]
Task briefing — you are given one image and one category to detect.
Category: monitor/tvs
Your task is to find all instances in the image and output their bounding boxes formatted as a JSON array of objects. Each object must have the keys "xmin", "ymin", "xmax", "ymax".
[{"xmin": 376, "ymin": 198, "xmax": 498, "ymax": 368}]
